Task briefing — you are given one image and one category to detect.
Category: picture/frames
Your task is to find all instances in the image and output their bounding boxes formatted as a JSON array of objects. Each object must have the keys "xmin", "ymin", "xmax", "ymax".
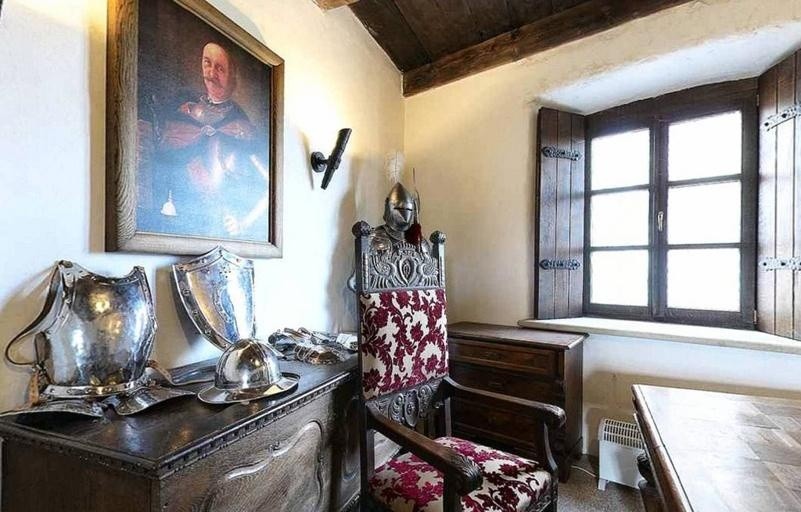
[{"xmin": 105, "ymin": 0, "xmax": 287, "ymax": 258}]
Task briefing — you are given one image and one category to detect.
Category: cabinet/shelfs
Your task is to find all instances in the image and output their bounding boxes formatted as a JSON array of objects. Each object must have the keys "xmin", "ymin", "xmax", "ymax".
[
  {"xmin": 626, "ymin": 381, "xmax": 801, "ymax": 511},
  {"xmin": 1, "ymin": 352, "xmax": 403, "ymax": 512},
  {"xmin": 444, "ymin": 321, "xmax": 590, "ymax": 484}
]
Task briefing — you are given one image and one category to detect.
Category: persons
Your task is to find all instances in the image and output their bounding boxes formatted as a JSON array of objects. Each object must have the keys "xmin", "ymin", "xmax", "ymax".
[{"xmin": 155, "ymin": 44, "xmax": 269, "ymax": 238}]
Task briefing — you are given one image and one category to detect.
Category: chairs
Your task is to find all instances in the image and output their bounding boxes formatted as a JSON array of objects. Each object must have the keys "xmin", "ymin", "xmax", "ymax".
[{"xmin": 349, "ymin": 220, "xmax": 569, "ymax": 512}]
